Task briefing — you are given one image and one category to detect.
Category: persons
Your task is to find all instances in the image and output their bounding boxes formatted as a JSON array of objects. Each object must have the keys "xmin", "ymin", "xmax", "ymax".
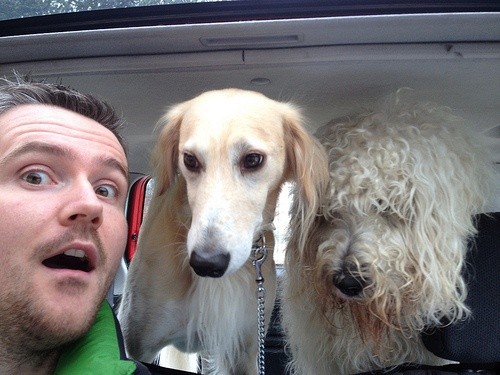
[{"xmin": 0, "ymin": 67, "xmax": 129, "ymax": 375}]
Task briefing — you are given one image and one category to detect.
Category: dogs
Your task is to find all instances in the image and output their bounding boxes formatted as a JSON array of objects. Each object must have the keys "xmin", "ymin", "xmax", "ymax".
[
  {"xmin": 279, "ymin": 88, "xmax": 495, "ymax": 375},
  {"xmin": 116, "ymin": 88, "xmax": 328, "ymax": 375}
]
